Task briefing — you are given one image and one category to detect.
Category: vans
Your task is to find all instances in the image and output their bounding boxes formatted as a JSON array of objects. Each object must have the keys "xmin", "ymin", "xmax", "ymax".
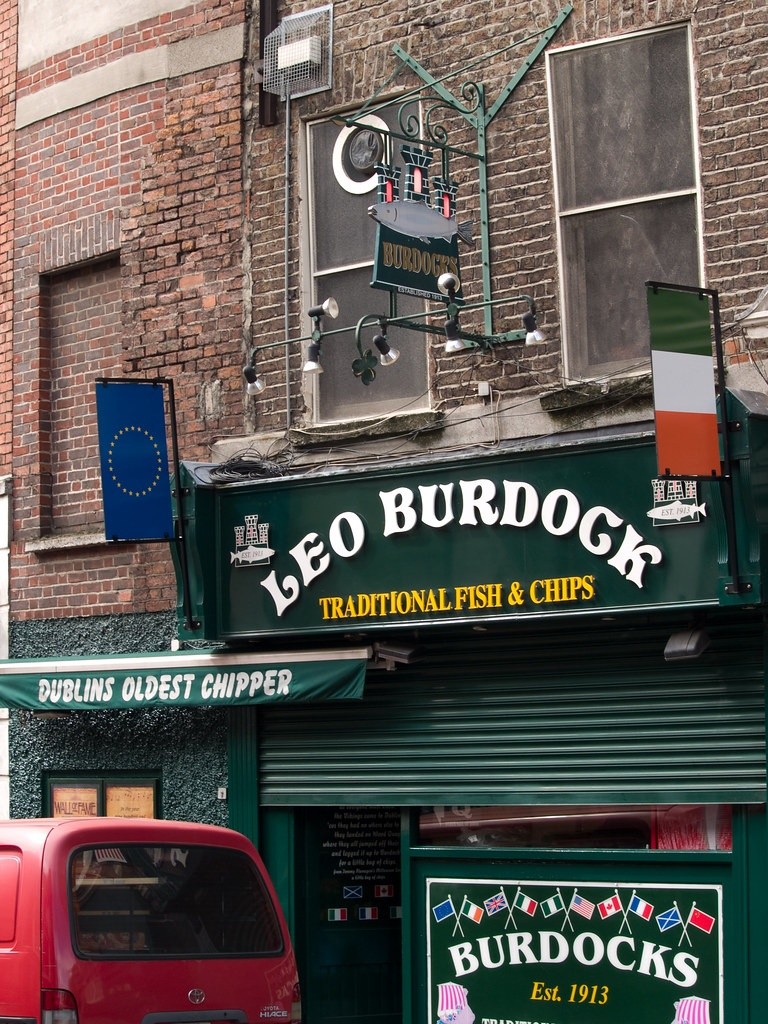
[{"xmin": 0, "ymin": 817, "xmax": 305, "ymax": 1023}]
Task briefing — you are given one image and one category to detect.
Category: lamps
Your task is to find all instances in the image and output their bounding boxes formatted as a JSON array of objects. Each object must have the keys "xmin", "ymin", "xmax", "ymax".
[
  {"xmin": 444, "ymin": 320, "xmax": 466, "ymax": 353},
  {"xmin": 373, "ymin": 334, "xmax": 400, "ymax": 365},
  {"xmin": 375, "ymin": 639, "xmax": 429, "ymax": 663},
  {"xmin": 242, "ymin": 366, "xmax": 266, "ymax": 396},
  {"xmin": 522, "ymin": 312, "xmax": 547, "ymax": 346},
  {"xmin": 436, "ymin": 273, "xmax": 461, "ymax": 303},
  {"xmin": 663, "ymin": 620, "xmax": 710, "ymax": 662},
  {"xmin": 303, "ymin": 343, "xmax": 325, "ymax": 375},
  {"xmin": 307, "ymin": 297, "xmax": 339, "ymax": 326}
]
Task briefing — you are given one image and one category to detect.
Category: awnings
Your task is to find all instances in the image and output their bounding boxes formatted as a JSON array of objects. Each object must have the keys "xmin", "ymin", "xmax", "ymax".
[{"xmin": 0, "ymin": 644, "xmax": 409, "ymax": 710}]
